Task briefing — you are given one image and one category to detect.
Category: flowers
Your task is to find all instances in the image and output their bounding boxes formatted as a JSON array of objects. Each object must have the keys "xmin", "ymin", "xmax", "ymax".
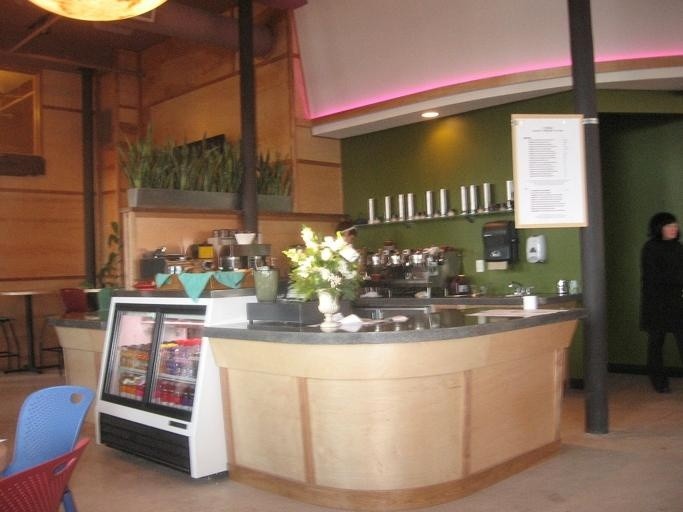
[{"xmin": 281, "ymin": 223, "xmax": 365, "ymax": 303}]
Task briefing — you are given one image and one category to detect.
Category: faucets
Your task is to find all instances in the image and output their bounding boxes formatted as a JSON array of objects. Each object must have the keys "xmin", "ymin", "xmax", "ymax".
[{"xmin": 506, "ymin": 281, "xmax": 525, "ymax": 294}]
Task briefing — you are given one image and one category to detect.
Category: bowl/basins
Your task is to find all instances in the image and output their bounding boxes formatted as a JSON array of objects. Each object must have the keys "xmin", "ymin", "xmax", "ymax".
[{"xmin": 233, "ymin": 232, "xmax": 256, "ymax": 245}]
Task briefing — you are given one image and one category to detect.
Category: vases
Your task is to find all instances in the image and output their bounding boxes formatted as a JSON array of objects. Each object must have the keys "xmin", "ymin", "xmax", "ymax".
[{"xmin": 317, "ymin": 293, "xmax": 342, "ymax": 332}]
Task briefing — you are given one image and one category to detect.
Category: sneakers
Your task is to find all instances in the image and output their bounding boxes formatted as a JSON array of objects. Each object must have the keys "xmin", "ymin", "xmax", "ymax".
[{"xmin": 654, "ymin": 375, "xmax": 669, "ymax": 393}]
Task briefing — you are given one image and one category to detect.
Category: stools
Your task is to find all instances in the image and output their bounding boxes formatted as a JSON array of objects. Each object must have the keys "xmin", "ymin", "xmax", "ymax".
[{"xmin": 0, "ymin": 317, "xmax": 21, "ymax": 370}]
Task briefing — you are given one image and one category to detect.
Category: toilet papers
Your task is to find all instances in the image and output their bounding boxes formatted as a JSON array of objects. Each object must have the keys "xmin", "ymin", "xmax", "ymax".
[{"xmin": 522, "ymin": 295, "xmax": 538, "ymax": 312}]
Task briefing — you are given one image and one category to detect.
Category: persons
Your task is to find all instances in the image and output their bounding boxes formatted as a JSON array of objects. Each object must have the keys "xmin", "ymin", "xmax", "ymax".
[
  {"xmin": 334, "ymin": 221, "xmax": 358, "ymax": 244},
  {"xmin": 636, "ymin": 212, "xmax": 682, "ymax": 392}
]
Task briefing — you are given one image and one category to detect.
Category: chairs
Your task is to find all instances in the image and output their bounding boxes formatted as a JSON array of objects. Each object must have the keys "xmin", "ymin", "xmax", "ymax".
[
  {"xmin": 0, "ymin": 384, "xmax": 96, "ymax": 512},
  {"xmin": 59, "ymin": 286, "xmax": 114, "ymax": 312}
]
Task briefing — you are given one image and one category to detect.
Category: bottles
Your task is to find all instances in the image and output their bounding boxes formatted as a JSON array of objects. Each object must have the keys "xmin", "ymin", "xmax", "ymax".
[{"xmin": 116, "ymin": 337, "xmax": 203, "ymax": 413}]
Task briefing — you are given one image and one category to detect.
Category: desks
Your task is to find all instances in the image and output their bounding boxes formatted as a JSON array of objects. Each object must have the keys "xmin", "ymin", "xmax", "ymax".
[{"xmin": 0, "ymin": 289, "xmax": 103, "ymax": 374}]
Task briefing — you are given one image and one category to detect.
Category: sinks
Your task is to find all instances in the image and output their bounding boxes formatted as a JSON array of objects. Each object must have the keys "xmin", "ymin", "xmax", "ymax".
[{"xmin": 505, "ymin": 292, "xmax": 533, "ymax": 296}]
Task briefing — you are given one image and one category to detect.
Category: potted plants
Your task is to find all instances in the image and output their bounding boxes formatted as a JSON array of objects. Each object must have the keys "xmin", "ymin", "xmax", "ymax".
[{"xmin": 116, "ymin": 119, "xmax": 293, "ymax": 214}]
